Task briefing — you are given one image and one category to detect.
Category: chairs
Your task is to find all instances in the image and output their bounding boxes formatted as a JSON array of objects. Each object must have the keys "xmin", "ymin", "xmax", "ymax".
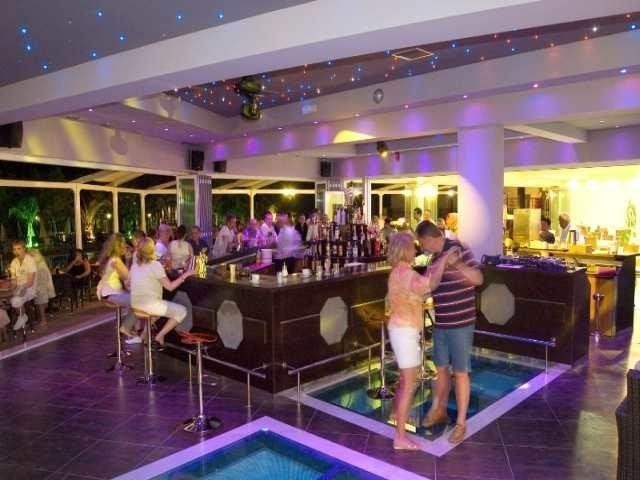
[{"xmin": 0, "ymin": 259, "xmax": 104, "ymax": 341}]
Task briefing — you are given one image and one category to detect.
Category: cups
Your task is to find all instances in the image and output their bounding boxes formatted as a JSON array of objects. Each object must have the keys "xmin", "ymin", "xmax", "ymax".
[
  {"xmin": 251, "ymin": 274, "xmax": 260, "ymax": 281},
  {"xmin": 316, "ymin": 259, "xmax": 339, "ymax": 277},
  {"xmin": 261, "ymin": 249, "xmax": 273, "ymax": 263},
  {"xmin": 608, "ymin": 239, "xmax": 618, "ymax": 256},
  {"xmin": 228, "ymin": 264, "xmax": 240, "ymax": 282},
  {"xmin": 567, "ymin": 261, "xmax": 575, "ymax": 270},
  {"xmin": 276, "ymin": 271, "xmax": 283, "ymax": 283}
]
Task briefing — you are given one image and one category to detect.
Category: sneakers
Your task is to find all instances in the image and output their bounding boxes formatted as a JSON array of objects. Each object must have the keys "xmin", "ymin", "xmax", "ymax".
[
  {"xmin": 420, "ymin": 405, "xmax": 450, "ymax": 429},
  {"xmin": 36, "ymin": 318, "xmax": 49, "ymax": 329},
  {"xmin": 448, "ymin": 422, "xmax": 468, "ymax": 445},
  {"xmin": 12, "ymin": 313, "xmax": 28, "ymax": 331},
  {"xmin": 393, "ymin": 440, "xmax": 423, "ymax": 452},
  {"xmin": 389, "ymin": 411, "xmax": 419, "ymax": 426},
  {"xmin": 119, "ymin": 325, "xmax": 144, "ymax": 345}
]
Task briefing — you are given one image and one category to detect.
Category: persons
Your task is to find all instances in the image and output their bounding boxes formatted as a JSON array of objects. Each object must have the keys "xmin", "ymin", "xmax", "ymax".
[
  {"xmin": 381, "ymin": 233, "xmax": 461, "ymax": 450},
  {"xmin": 153, "ymin": 208, "xmax": 441, "ymax": 276},
  {"xmin": 414, "ymin": 220, "xmax": 485, "ymax": 444},
  {"xmin": 0, "ymin": 240, "xmax": 36, "ymax": 330},
  {"xmin": 96, "ymin": 233, "xmax": 137, "ymax": 337},
  {"xmin": 127, "ymin": 238, "xmax": 195, "ymax": 348},
  {"xmin": 558, "ymin": 213, "xmax": 585, "ymax": 248},
  {"xmin": 29, "ymin": 247, "xmax": 57, "ymax": 328},
  {"xmin": 540, "ymin": 217, "xmax": 554, "ymax": 244},
  {"xmin": 60, "ymin": 250, "xmax": 92, "ymax": 314}
]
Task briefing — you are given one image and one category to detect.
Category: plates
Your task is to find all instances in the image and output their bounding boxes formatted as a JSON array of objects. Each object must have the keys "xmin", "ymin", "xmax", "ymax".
[{"xmin": 248, "ymin": 279, "xmax": 263, "ymax": 285}]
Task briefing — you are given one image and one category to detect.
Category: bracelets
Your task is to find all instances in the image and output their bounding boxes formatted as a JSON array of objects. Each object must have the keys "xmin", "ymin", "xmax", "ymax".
[{"xmin": 181, "ymin": 273, "xmax": 185, "ymax": 283}]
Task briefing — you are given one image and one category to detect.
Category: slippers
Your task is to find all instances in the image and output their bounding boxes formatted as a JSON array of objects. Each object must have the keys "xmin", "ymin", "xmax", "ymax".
[{"xmin": 151, "ymin": 342, "xmax": 169, "ymax": 349}]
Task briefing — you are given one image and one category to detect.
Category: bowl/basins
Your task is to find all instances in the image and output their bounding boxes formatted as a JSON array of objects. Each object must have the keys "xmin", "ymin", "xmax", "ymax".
[{"xmin": 302, "ymin": 269, "xmax": 312, "ymax": 278}]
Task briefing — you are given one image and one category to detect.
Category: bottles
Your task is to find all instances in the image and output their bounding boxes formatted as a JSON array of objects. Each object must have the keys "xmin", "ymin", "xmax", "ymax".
[
  {"xmin": 312, "ymin": 223, "xmax": 366, "ymax": 257},
  {"xmin": 312, "ymin": 257, "xmax": 322, "ymax": 271},
  {"xmin": 336, "ymin": 208, "xmax": 346, "ymax": 225},
  {"xmin": 367, "ymin": 233, "xmax": 384, "ymax": 257},
  {"xmin": 256, "ymin": 249, "xmax": 261, "ymax": 264},
  {"xmin": 281, "ymin": 261, "xmax": 289, "ymax": 276},
  {"xmin": 303, "ymin": 247, "xmax": 312, "ymax": 267}
]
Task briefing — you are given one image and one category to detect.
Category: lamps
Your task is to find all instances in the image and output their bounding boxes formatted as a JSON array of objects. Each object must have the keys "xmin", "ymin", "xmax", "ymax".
[{"xmin": 376, "ymin": 140, "xmax": 390, "ymax": 160}]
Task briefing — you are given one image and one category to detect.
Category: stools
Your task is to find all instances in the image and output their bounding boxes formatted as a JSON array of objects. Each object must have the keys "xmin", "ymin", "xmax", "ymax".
[
  {"xmin": 175, "ymin": 325, "xmax": 225, "ymax": 434},
  {"xmin": 416, "ymin": 302, "xmax": 439, "ymax": 380},
  {"xmin": 363, "ymin": 305, "xmax": 401, "ymax": 400},
  {"xmin": 130, "ymin": 306, "xmax": 169, "ymax": 388},
  {"xmin": 589, "ymin": 266, "xmax": 617, "ymax": 336},
  {"xmin": 103, "ymin": 297, "xmax": 135, "ymax": 374}
]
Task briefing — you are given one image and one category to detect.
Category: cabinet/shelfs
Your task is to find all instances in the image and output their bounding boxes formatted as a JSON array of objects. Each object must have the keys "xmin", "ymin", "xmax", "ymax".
[{"xmin": 301, "ymin": 221, "xmax": 386, "ymax": 273}]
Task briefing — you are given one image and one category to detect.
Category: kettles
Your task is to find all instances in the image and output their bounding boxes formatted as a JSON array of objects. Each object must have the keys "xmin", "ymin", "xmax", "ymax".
[{"xmin": 569, "ymin": 229, "xmax": 579, "ymax": 245}]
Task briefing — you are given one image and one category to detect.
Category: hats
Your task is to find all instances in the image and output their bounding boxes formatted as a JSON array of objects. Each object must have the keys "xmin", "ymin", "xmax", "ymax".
[{"xmin": 158, "ymin": 223, "xmax": 170, "ymax": 233}]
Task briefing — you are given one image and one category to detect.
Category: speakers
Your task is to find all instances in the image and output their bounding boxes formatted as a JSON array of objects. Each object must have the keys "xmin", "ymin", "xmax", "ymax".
[
  {"xmin": 189, "ymin": 150, "xmax": 204, "ymax": 170},
  {"xmin": 0, "ymin": 121, "xmax": 23, "ymax": 148},
  {"xmin": 214, "ymin": 160, "xmax": 226, "ymax": 172},
  {"xmin": 320, "ymin": 161, "xmax": 330, "ymax": 177}
]
